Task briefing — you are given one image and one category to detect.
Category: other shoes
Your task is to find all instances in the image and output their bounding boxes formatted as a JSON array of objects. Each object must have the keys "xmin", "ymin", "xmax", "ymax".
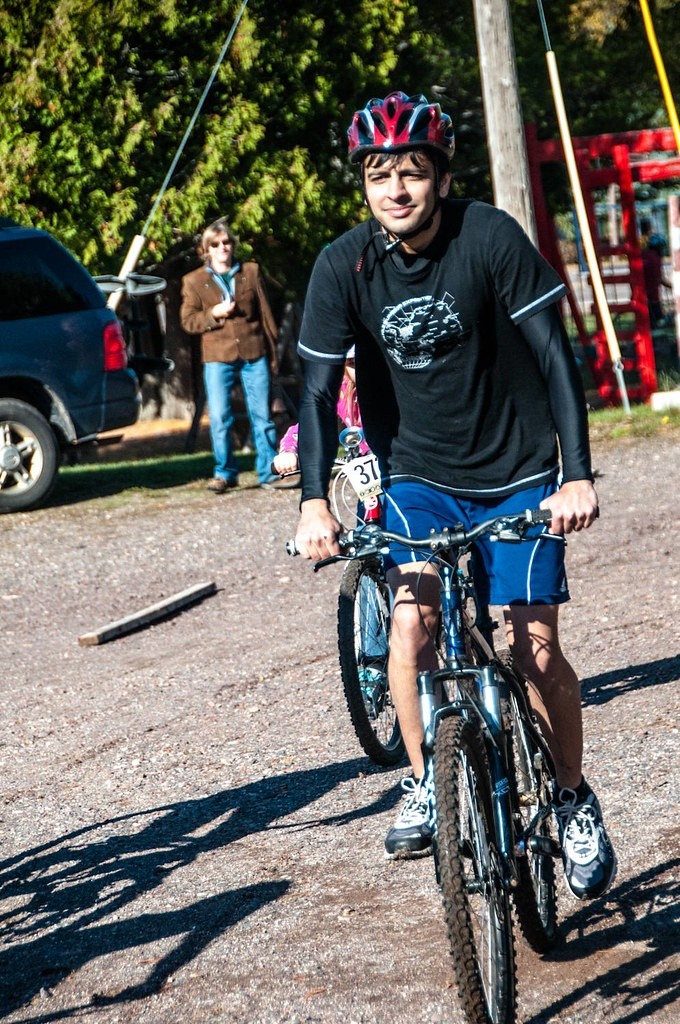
[
  {"xmin": 261, "ymin": 473, "xmax": 301, "ymax": 491},
  {"xmin": 207, "ymin": 478, "xmax": 239, "ymax": 492}
]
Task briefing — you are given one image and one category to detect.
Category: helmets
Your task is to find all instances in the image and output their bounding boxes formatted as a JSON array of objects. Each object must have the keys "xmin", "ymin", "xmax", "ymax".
[{"xmin": 346, "ymin": 92, "xmax": 455, "ymax": 164}]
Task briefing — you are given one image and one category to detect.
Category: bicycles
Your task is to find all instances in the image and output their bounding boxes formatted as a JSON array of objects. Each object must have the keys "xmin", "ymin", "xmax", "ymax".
[{"xmin": 283, "ymin": 420, "xmax": 601, "ymax": 1024}]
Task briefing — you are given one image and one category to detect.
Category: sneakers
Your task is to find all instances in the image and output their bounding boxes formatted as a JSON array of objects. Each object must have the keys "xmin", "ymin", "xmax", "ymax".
[
  {"xmin": 356, "ymin": 651, "xmax": 387, "ymax": 719},
  {"xmin": 552, "ymin": 788, "xmax": 617, "ymax": 900},
  {"xmin": 384, "ymin": 778, "xmax": 434, "ymax": 861}
]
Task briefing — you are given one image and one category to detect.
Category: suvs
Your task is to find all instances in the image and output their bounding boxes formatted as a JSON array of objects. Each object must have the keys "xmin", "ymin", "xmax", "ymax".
[{"xmin": 0, "ymin": 213, "xmax": 143, "ymax": 515}]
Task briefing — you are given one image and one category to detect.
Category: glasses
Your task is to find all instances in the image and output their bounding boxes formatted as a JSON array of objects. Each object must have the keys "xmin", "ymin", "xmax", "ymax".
[{"xmin": 208, "ymin": 240, "xmax": 231, "ymax": 248}]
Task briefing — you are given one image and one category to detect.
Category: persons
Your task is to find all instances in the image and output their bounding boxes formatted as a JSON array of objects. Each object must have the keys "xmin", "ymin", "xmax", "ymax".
[
  {"xmin": 179, "ymin": 218, "xmax": 280, "ymax": 492},
  {"xmin": 273, "ymin": 93, "xmax": 623, "ymax": 898},
  {"xmin": 638, "ymin": 219, "xmax": 672, "ymax": 326}
]
